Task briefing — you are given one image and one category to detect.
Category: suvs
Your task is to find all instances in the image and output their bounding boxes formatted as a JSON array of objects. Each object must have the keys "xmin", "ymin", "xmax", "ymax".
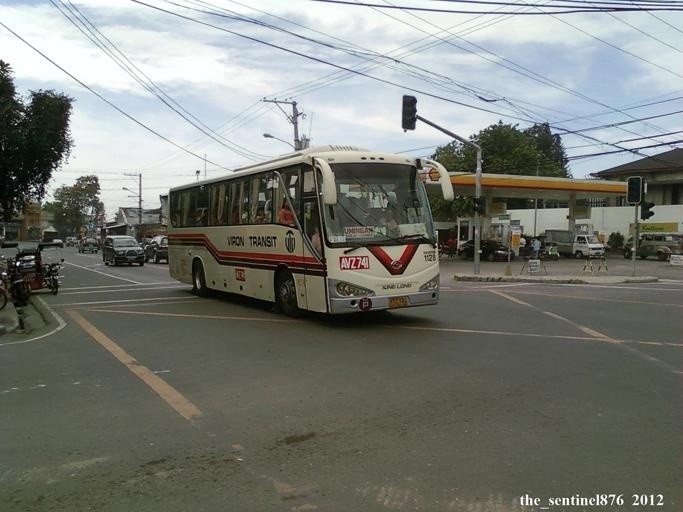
[{"xmin": 101, "ymin": 235, "xmax": 144, "ymax": 266}]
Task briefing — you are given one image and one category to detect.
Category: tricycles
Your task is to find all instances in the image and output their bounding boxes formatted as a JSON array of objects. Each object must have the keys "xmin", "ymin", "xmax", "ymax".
[{"xmin": 522, "ymin": 235, "xmax": 560, "ymax": 261}]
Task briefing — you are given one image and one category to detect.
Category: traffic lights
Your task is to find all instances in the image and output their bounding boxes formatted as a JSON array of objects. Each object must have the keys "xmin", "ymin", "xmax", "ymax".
[
  {"xmin": 472, "ymin": 197, "xmax": 486, "ymax": 216},
  {"xmin": 626, "ymin": 176, "xmax": 643, "ymax": 205},
  {"xmin": 640, "ymin": 200, "xmax": 654, "ymax": 220},
  {"xmin": 401, "ymin": 95, "xmax": 417, "ymax": 129}
]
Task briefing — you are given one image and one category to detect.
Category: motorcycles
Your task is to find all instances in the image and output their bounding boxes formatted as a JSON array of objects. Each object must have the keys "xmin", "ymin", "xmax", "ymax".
[
  {"xmin": 39, "ymin": 258, "xmax": 64, "ymax": 294},
  {"xmin": 7, "ymin": 260, "xmax": 31, "ymax": 305}
]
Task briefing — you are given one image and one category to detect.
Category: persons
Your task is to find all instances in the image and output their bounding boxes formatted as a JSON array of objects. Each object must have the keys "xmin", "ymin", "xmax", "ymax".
[{"xmin": 187, "ymin": 201, "xmax": 297, "ymax": 227}]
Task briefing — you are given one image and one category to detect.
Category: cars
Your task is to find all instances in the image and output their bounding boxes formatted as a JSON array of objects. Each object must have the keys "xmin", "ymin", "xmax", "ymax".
[
  {"xmin": 77, "ymin": 236, "xmax": 99, "ymax": 253},
  {"xmin": 457, "ymin": 239, "xmax": 515, "ymax": 262},
  {"xmin": 143, "ymin": 236, "xmax": 168, "ymax": 263}
]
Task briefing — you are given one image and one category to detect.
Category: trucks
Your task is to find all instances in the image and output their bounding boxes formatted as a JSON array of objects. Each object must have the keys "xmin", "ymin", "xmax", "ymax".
[{"xmin": 541, "ymin": 229, "xmax": 604, "ymax": 259}]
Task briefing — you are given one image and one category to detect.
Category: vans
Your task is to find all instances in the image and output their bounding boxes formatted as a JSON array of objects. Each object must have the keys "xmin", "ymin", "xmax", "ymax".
[{"xmin": 623, "ymin": 231, "xmax": 682, "ymax": 261}]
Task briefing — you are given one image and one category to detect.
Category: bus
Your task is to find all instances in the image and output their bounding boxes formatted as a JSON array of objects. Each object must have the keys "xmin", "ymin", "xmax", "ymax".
[{"xmin": 165, "ymin": 144, "xmax": 455, "ymax": 317}]
